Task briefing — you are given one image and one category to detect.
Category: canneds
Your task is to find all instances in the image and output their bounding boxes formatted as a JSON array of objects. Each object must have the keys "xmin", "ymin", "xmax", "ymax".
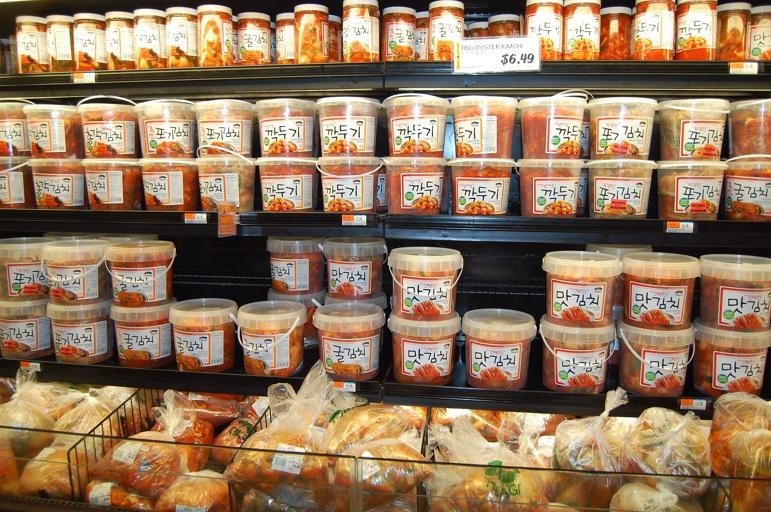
[
  {"xmin": 343, "ymin": 0, "xmax": 381, "ymax": 63},
  {"xmin": 71, "ymin": 13, "xmax": 104, "ymax": 73},
  {"xmin": 429, "ymin": 0, "xmax": 464, "ymax": 63},
  {"xmin": 134, "ymin": 9, "xmax": 165, "ymax": 67},
  {"xmin": 719, "ymin": 3, "xmax": 752, "ymax": 60},
  {"xmin": 467, "ymin": 22, "xmax": 488, "ymax": 39},
  {"xmin": 271, "ymin": 22, "xmax": 275, "ymax": 63},
  {"xmin": 676, "ymin": 0, "xmax": 720, "ymax": 60},
  {"xmin": 563, "ymin": 0, "xmax": 601, "ymax": 59},
  {"xmin": 46, "ymin": 15, "xmax": 72, "ymax": 73},
  {"xmin": 15, "ymin": 15, "xmax": 49, "ymax": 74},
  {"xmin": 294, "ymin": 3, "xmax": 331, "ymax": 63},
  {"xmin": 332, "ymin": 13, "xmax": 343, "ymax": 61},
  {"xmin": 238, "ymin": 12, "xmax": 270, "ymax": 67},
  {"xmin": 632, "ymin": 3, "xmax": 676, "ymax": 62},
  {"xmin": 166, "ymin": 6, "xmax": 198, "ymax": 70},
  {"xmin": 746, "ymin": 4, "xmax": 771, "ymax": 58},
  {"xmin": 276, "ymin": 13, "xmax": 297, "ymax": 64},
  {"xmin": 601, "ymin": 5, "xmax": 632, "ymax": 61},
  {"xmin": 195, "ymin": 4, "xmax": 233, "ymax": 68},
  {"xmin": 488, "ymin": 13, "xmax": 522, "ymax": 36},
  {"xmin": 104, "ymin": 10, "xmax": 134, "ymax": 74},
  {"xmin": 232, "ymin": 15, "xmax": 237, "ymax": 63},
  {"xmin": 418, "ymin": 10, "xmax": 429, "ymax": 62},
  {"xmin": 381, "ymin": 6, "xmax": 417, "ymax": 61},
  {"xmin": 526, "ymin": 0, "xmax": 562, "ymax": 60}
]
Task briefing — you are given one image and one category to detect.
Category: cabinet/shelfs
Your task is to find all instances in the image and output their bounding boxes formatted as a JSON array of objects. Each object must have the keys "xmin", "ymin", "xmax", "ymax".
[{"xmin": 1, "ymin": 4, "xmax": 771, "ymax": 512}]
